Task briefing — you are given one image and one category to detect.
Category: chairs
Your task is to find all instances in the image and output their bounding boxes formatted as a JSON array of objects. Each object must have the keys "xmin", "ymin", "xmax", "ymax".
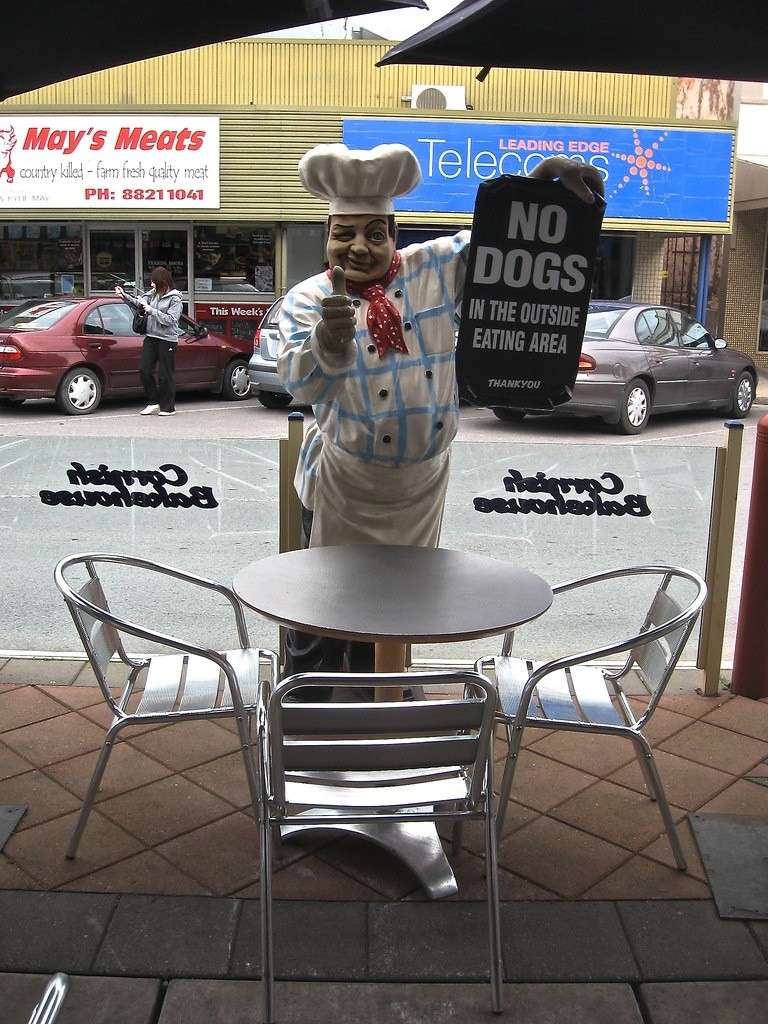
[
  {"xmin": 252, "ymin": 673, "xmax": 506, "ymax": 1024},
  {"xmin": 54, "ymin": 553, "xmax": 280, "ymax": 865},
  {"xmin": 454, "ymin": 565, "xmax": 709, "ymax": 887}
]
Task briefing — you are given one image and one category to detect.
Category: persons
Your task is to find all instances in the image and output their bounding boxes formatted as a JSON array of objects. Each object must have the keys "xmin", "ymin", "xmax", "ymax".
[
  {"xmin": 278, "ymin": 146, "xmax": 603, "ymax": 704},
  {"xmin": 115, "ymin": 267, "xmax": 183, "ymax": 416}
]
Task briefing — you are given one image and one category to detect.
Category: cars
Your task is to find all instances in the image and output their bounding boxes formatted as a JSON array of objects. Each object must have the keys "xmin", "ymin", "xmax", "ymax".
[
  {"xmin": 488, "ymin": 301, "xmax": 758, "ymax": 436},
  {"xmin": 172, "ymin": 275, "xmax": 260, "ymax": 293},
  {"xmin": 0, "ymin": 296, "xmax": 255, "ymax": 415},
  {"xmin": 249, "ymin": 296, "xmax": 485, "ymax": 408},
  {"xmin": 0, "ymin": 271, "xmax": 78, "ymax": 299}
]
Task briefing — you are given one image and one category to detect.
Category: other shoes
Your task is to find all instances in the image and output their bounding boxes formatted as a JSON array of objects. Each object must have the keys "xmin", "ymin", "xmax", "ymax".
[
  {"xmin": 159, "ymin": 410, "xmax": 176, "ymax": 416},
  {"xmin": 140, "ymin": 404, "xmax": 160, "ymax": 415}
]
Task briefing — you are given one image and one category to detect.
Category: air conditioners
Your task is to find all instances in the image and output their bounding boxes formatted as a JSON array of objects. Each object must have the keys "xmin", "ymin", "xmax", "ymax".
[{"xmin": 411, "ymin": 86, "xmax": 468, "ymax": 112}]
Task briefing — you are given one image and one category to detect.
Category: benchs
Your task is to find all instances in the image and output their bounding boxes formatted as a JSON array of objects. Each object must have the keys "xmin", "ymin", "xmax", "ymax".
[{"xmin": 232, "ymin": 544, "xmax": 555, "ymax": 900}]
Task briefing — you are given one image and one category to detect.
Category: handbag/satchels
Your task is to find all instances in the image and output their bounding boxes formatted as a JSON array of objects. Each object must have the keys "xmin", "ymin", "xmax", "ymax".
[{"xmin": 118, "ymin": 287, "xmax": 149, "ymax": 334}]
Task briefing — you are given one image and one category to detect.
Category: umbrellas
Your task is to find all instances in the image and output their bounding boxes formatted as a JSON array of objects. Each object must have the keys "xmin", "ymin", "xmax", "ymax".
[
  {"xmin": 376, "ymin": 0, "xmax": 768, "ymax": 82},
  {"xmin": 0, "ymin": 0, "xmax": 430, "ymax": 103}
]
